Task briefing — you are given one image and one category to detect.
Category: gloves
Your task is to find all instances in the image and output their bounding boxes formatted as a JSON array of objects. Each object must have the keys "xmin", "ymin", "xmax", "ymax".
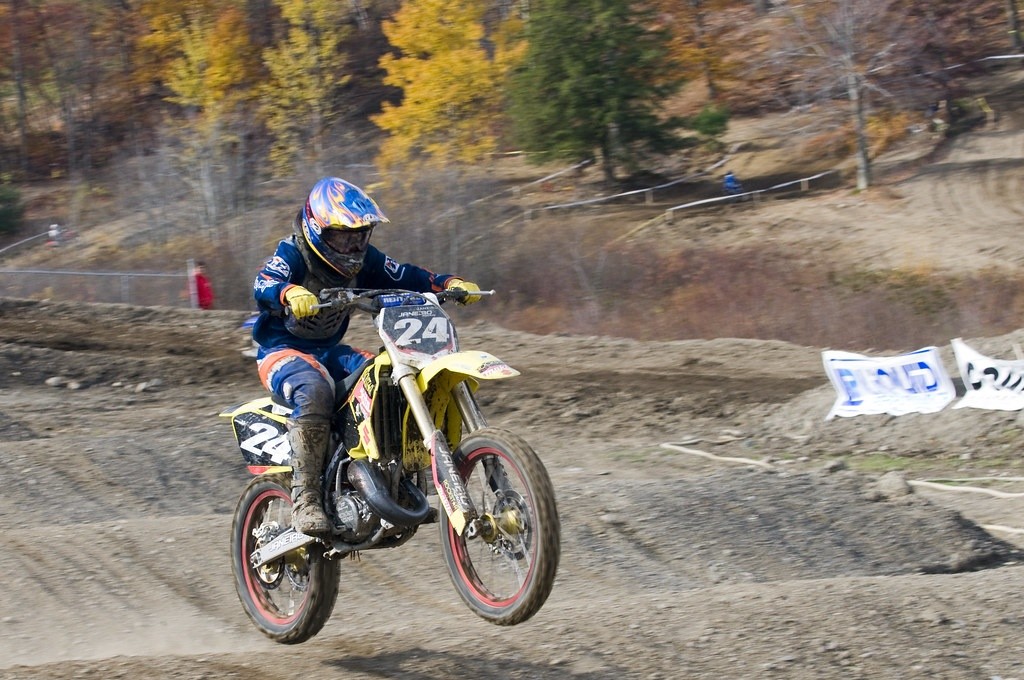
[
  {"xmin": 448, "ymin": 278, "xmax": 482, "ymax": 308},
  {"xmin": 286, "ymin": 285, "xmax": 320, "ymax": 320}
]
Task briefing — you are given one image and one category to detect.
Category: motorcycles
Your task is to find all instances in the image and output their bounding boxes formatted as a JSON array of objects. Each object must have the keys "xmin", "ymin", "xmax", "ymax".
[{"xmin": 219, "ymin": 286, "xmax": 562, "ymax": 646}]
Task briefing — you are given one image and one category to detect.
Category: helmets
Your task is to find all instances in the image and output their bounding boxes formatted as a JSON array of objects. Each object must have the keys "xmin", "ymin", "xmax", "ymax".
[{"xmin": 292, "ymin": 176, "xmax": 391, "ymax": 287}]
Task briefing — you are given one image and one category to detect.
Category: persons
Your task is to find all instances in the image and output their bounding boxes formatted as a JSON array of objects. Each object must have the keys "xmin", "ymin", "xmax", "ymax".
[
  {"xmin": 189, "ymin": 261, "xmax": 216, "ymax": 309},
  {"xmin": 48, "ymin": 224, "xmax": 63, "ymax": 239},
  {"xmin": 251, "ymin": 177, "xmax": 482, "ymax": 535},
  {"xmin": 723, "ymin": 171, "xmax": 740, "ymax": 188}
]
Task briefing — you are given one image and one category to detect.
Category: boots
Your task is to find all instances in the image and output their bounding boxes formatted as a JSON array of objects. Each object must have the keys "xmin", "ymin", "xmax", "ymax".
[
  {"xmin": 422, "ymin": 506, "xmax": 439, "ymax": 524},
  {"xmin": 286, "ymin": 418, "xmax": 329, "ymax": 532}
]
